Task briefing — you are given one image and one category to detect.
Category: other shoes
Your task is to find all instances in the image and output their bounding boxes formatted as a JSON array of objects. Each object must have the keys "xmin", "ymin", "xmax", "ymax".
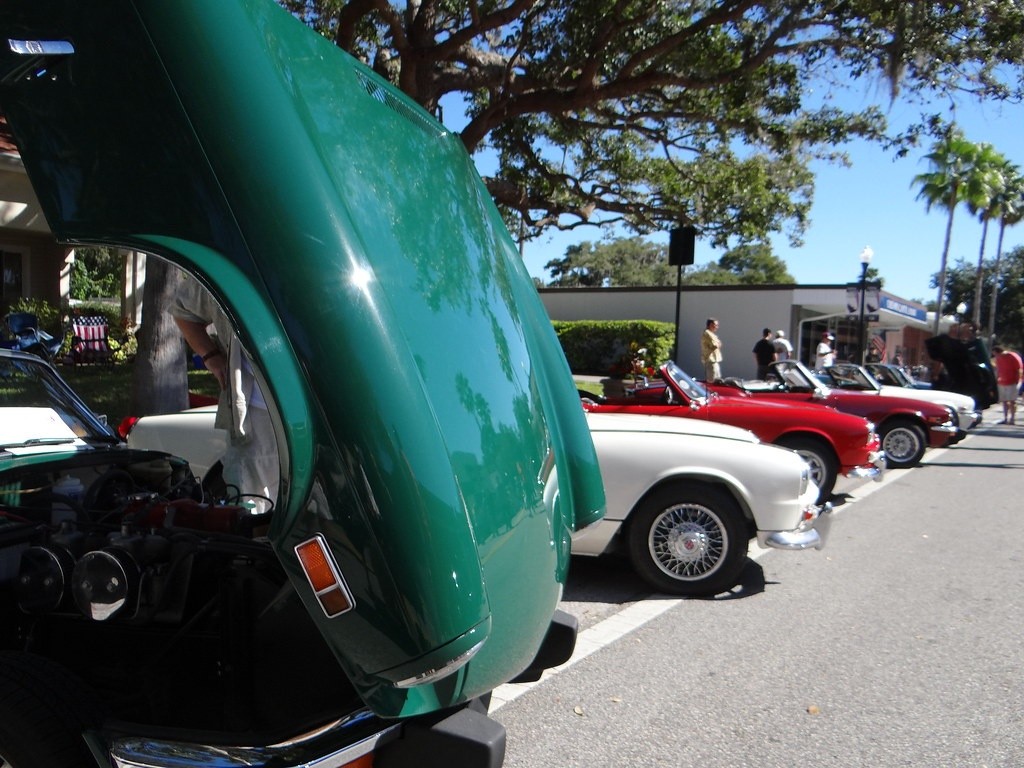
[
  {"xmin": 1009, "ymin": 417, "xmax": 1015, "ymax": 426},
  {"xmin": 997, "ymin": 419, "xmax": 1008, "ymax": 425}
]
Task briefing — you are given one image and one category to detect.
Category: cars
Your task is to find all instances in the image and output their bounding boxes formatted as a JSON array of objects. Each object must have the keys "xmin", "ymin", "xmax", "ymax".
[
  {"xmin": 118, "ymin": 401, "xmax": 828, "ymax": 600},
  {"xmin": 0, "ymin": 0, "xmax": 607, "ymax": 768},
  {"xmin": 635, "ymin": 332, "xmax": 1000, "ymax": 470},
  {"xmin": 577, "ymin": 360, "xmax": 886, "ymax": 511}
]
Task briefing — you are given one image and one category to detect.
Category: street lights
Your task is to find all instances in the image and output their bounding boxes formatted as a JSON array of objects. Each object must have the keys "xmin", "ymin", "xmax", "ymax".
[
  {"xmin": 957, "ymin": 300, "xmax": 969, "ymax": 335},
  {"xmin": 859, "ymin": 246, "xmax": 872, "ymax": 358}
]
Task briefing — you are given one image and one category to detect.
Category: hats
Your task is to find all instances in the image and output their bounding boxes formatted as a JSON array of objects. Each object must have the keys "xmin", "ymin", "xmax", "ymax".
[
  {"xmin": 823, "ymin": 332, "xmax": 834, "ymax": 341},
  {"xmin": 775, "ymin": 330, "xmax": 784, "ymax": 337}
]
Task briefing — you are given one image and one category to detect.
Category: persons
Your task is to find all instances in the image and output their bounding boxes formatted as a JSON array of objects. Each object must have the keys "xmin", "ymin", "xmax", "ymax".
[
  {"xmin": 866, "ymin": 345, "xmax": 882, "ymax": 374},
  {"xmin": 752, "ymin": 327, "xmax": 777, "ymax": 380},
  {"xmin": 771, "ymin": 329, "xmax": 792, "ymax": 371},
  {"xmin": 168, "ymin": 272, "xmax": 280, "ymax": 516},
  {"xmin": 701, "ymin": 318, "xmax": 723, "ymax": 384},
  {"xmin": 990, "ymin": 346, "xmax": 1023, "ymax": 425},
  {"xmin": 815, "ymin": 332, "xmax": 837, "ymax": 374}
]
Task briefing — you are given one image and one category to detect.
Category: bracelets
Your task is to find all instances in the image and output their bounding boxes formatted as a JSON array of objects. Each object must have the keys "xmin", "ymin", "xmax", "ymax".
[{"xmin": 199, "ymin": 347, "xmax": 220, "ymax": 363}]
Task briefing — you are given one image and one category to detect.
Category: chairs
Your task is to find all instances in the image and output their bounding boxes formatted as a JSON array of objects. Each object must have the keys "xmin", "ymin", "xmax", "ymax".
[
  {"xmin": 60, "ymin": 315, "xmax": 123, "ymax": 373},
  {"xmin": 0, "ymin": 312, "xmax": 60, "ymax": 382}
]
